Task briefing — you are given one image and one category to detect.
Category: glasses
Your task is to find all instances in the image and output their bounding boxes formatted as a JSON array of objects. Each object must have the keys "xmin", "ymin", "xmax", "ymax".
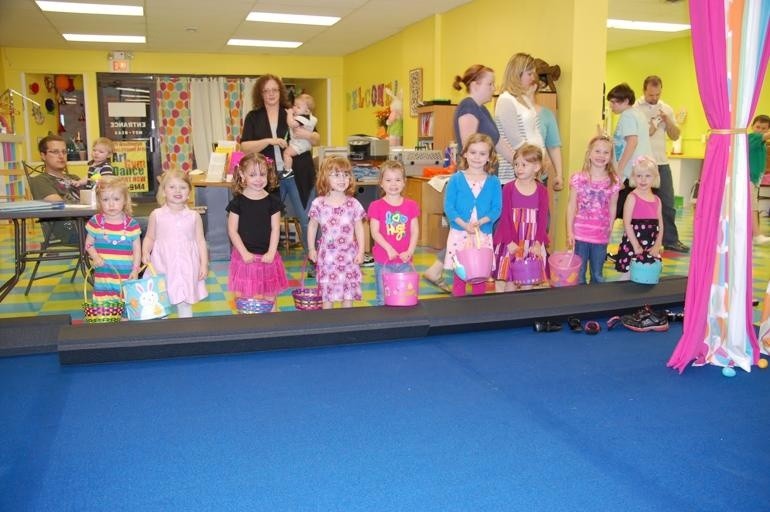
[
  {"xmin": 329, "ymin": 173, "xmax": 349, "ymax": 179},
  {"xmin": 45, "ymin": 149, "xmax": 68, "ymax": 154},
  {"xmin": 261, "ymin": 87, "xmax": 282, "ymax": 94}
]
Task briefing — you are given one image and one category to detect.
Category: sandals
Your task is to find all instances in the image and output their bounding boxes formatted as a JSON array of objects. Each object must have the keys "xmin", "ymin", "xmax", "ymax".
[
  {"xmin": 534, "ymin": 320, "xmax": 557, "ymax": 332},
  {"xmin": 569, "ymin": 317, "xmax": 583, "ymax": 333}
]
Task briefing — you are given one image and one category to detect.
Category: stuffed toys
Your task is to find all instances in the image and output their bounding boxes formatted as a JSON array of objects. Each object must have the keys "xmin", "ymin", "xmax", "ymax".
[{"xmin": 385, "ymin": 88, "xmax": 403, "ymax": 146}]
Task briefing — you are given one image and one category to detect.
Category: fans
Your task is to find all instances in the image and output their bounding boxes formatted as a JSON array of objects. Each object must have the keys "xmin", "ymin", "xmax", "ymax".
[{"xmin": 535, "ymin": 59, "xmax": 561, "ymax": 91}]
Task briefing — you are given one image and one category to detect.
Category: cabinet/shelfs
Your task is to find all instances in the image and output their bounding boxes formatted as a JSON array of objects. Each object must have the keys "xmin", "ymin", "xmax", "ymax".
[
  {"xmin": 492, "ymin": 91, "xmax": 556, "ymax": 118},
  {"xmin": 281, "ymin": 78, "xmax": 328, "ymax": 156},
  {"xmin": 401, "ymin": 104, "xmax": 458, "ymax": 247}
]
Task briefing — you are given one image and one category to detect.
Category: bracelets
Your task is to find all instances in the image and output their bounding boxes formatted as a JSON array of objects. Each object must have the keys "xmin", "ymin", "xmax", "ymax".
[
  {"xmin": 554, "ymin": 174, "xmax": 563, "ymax": 179},
  {"xmin": 307, "ymin": 246, "xmax": 315, "ymax": 250}
]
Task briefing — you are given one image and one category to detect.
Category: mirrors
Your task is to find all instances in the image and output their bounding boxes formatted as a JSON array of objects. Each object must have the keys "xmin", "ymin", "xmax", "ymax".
[
  {"xmin": 21, "ymin": 70, "xmax": 93, "ymax": 166},
  {"xmin": 96, "ymin": 72, "xmax": 160, "ymax": 202}
]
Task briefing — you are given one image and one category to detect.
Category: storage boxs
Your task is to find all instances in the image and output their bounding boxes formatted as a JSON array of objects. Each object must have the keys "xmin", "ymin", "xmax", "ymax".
[
  {"xmin": 280, "ymin": 223, "xmax": 301, "ymax": 242},
  {"xmin": 392, "ymin": 146, "xmax": 443, "ymax": 177}
]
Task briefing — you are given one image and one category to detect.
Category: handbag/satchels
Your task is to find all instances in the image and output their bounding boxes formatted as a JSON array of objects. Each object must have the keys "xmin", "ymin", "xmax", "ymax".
[{"xmin": 123, "ymin": 264, "xmax": 171, "ymax": 321}]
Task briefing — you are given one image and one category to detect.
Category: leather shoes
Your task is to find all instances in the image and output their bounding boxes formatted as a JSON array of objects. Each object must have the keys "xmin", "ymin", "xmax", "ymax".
[{"xmin": 665, "ymin": 241, "xmax": 690, "ymax": 252}]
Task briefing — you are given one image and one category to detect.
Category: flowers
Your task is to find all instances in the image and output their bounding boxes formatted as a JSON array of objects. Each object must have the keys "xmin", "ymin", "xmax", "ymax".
[{"xmin": 375, "ymin": 107, "xmax": 391, "ymax": 139}]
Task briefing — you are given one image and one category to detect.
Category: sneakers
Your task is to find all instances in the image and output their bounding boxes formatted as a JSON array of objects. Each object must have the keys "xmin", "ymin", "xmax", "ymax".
[
  {"xmin": 279, "ymin": 169, "xmax": 294, "ymax": 179},
  {"xmin": 752, "ymin": 235, "xmax": 770, "ymax": 246},
  {"xmin": 623, "ymin": 308, "xmax": 668, "ymax": 332}
]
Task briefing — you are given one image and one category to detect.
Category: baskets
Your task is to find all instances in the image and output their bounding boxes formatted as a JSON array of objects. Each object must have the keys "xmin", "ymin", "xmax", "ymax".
[
  {"xmin": 292, "ymin": 258, "xmax": 323, "ymax": 311},
  {"xmin": 82, "ymin": 264, "xmax": 124, "ymax": 324},
  {"xmin": 234, "ymin": 258, "xmax": 275, "ymax": 315}
]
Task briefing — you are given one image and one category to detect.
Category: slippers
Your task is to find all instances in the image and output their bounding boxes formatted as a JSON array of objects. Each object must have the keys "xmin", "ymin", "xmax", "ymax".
[
  {"xmin": 608, "ymin": 317, "xmax": 622, "ymax": 331},
  {"xmin": 585, "ymin": 321, "xmax": 600, "ymax": 334}
]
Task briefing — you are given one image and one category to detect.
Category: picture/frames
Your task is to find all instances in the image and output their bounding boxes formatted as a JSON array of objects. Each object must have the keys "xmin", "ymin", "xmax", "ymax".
[{"xmin": 408, "ymin": 67, "xmax": 423, "ymax": 117}]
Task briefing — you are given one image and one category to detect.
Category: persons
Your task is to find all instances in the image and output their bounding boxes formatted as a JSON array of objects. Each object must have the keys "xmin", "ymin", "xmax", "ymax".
[
  {"xmin": 85, "ymin": 177, "xmax": 142, "ymax": 321},
  {"xmin": 422, "ymin": 64, "xmax": 501, "ymax": 293},
  {"xmin": 367, "ymin": 159, "xmax": 421, "ymax": 306},
  {"xmin": 279, "ymin": 94, "xmax": 318, "ymax": 180},
  {"xmin": 225, "ymin": 153, "xmax": 289, "ymax": 312},
  {"xmin": 306, "ymin": 154, "xmax": 367, "ymax": 310},
  {"xmin": 494, "ymin": 52, "xmax": 551, "ymax": 188},
  {"xmin": 606, "ymin": 84, "xmax": 661, "ymax": 264},
  {"xmin": 567, "ymin": 137, "xmax": 622, "ymax": 284},
  {"xmin": 137, "ymin": 170, "xmax": 209, "ymax": 318},
  {"xmin": 615, "ymin": 155, "xmax": 664, "ymax": 282},
  {"xmin": 239, "ymin": 74, "xmax": 320, "ymax": 278},
  {"xmin": 527, "ymin": 59, "xmax": 564, "ymax": 259},
  {"xmin": 632, "ymin": 76, "xmax": 690, "ymax": 252},
  {"xmin": 491, "ymin": 144, "xmax": 550, "ymax": 292},
  {"xmin": 443, "ymin": 133, "xmax": 502, "ymax": 297},
  {"xmin": 29, "ymin": 135, "xmax": 150, "ymax": 244},
  {"xmin": 747, "ymin": 115, "xmax": 770, "ymax": 245},
  {"xmin": 71, "ymin": 137, "xmax": 114, "ymax": 190}
]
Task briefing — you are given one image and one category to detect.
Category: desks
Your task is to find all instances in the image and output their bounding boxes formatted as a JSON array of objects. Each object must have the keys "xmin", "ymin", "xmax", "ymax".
[
  {"xmin": 319, "ymin": 149, "xmax": 379, "ymax": 209},
  {"xmin": 191, "ymin": 181, "xmax": 233, "ymax": 261},
  {"xmin": 668, "ymin": 157, "xmax": 704, "ymax": 206},
  {"xmin": 0, "ymin": 200, "xmax": 99, "ymax": 303}
]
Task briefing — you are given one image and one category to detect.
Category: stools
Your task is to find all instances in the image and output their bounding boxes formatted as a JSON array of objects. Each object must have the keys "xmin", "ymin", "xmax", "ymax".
[
  {"xmin": 755, "ymin": 185, "xmax": 770, "ymax": 224},
  {"xmin": 278, "ymin": 217, "xmax": 302, "ymax": 256}
]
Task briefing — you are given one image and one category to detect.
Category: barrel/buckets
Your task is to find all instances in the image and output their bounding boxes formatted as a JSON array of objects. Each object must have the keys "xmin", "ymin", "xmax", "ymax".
[
  {"xmin": 548, "ymin": 244, "xmax": 583, "ymax": 288},
  {"xmin": 455, "ymin": 226, "xmax": 494, "ymax": 285},
  {"xmin": 381, "ymin": 254, "xmax": 420, "ymax": 307}
]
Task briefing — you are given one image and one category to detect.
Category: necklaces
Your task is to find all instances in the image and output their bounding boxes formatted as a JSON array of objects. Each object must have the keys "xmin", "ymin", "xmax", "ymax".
[{"xmin": 100, "ymin": 212, "xmax": 128, "ymax": 245}]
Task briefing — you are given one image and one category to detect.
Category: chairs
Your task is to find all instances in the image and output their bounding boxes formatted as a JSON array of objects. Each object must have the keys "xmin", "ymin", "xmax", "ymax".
[{"xmin": 22, "ymin": 160, "xmax": 91, "ymax": 296}]
